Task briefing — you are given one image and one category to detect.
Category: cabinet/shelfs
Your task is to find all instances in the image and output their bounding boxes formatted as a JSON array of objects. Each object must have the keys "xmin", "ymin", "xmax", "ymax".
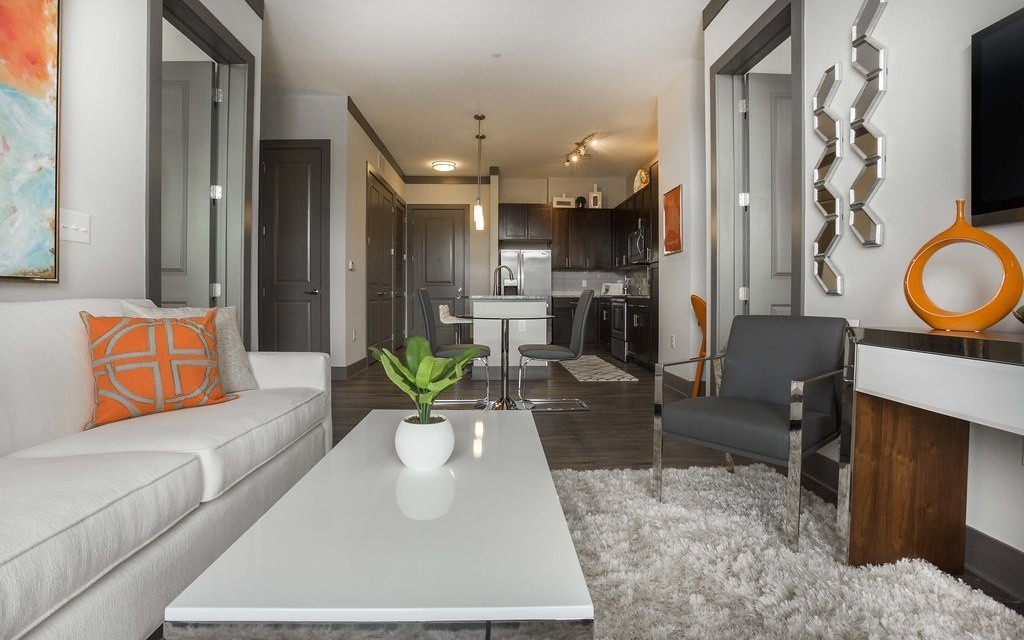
[
  {"xmin": 627, "ymin": 298, "xmax": 652, "ymax": 365},
  {"xmin": 498, "ymin": 202, "xmax": 552, "ymax": 242},
  {"xmin": 552, "ymin": 162, "xmax": 659, "ymax": 272},
  {"xmin": 552, "ymin": 296, "xmax": 612, "ymax": 346}
]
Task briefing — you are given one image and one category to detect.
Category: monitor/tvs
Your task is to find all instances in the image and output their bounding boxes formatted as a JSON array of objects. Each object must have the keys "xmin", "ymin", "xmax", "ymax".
[{"xmin": 970, "ymin": 7, "xmax": 1024, "ymax": 228}]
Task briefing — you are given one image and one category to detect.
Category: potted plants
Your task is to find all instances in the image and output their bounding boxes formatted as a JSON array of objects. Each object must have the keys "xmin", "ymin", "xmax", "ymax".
[{"xmin": 369, "ymin": 336, "xmax": 480, "ymax": 470}]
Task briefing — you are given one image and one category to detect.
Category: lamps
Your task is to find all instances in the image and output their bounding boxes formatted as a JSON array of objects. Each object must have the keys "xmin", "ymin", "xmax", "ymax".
[
  {"xmin": 565, "ymin": 133, "xmax": 597, "ymax": 167},
  {"xmin": 474, "ymin": 115, "xmax": 486, "ymax": 231},
  {"xmin": 433, "ymin": 161, "xmax": 455, "ymax": 172}
]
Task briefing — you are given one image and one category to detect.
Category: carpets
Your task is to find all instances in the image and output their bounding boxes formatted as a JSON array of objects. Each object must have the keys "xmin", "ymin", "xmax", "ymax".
[
  {"xmin": 165, "ymin": 463, "xmax": 1023, "ymax": 640},
  {"xmin": 560, "ymin": 355, "xmax": 639, "ymax": 382}
]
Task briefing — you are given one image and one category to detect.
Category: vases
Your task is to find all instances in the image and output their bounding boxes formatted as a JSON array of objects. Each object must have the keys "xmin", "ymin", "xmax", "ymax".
[{"xmin": 397, "ymin": 466, "xmax": 455, "ymax": 520}]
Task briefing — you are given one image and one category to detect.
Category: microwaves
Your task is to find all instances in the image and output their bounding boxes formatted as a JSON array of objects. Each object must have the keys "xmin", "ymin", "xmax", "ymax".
[{"xmin": 627, "ymin": 227, "xmax": 647, "ymax": 262}]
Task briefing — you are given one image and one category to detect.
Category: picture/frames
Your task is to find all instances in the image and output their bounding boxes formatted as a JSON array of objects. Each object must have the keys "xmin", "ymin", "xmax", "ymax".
[
  {"xmin": 0, "ymin": 1, "xmax": 63, "ymax": 283},
  {"xmin": 663, "ymin": 184, "xmax": 683, "ymax": 256}
]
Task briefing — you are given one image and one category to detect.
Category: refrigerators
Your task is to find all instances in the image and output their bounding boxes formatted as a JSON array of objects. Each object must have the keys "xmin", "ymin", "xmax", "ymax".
[{"xmin": 500, "ymin": 249, "xmax": 552, "ymax": 345}]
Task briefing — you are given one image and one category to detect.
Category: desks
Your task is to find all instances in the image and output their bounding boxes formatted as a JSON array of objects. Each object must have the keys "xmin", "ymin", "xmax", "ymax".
[
  {"xmin": 835, "ymin": 326, "xmax": 1024, "ymax": 575},
  {"xmin": 456, "ymin": 314, "xmax": 555, "ymax": 410}
]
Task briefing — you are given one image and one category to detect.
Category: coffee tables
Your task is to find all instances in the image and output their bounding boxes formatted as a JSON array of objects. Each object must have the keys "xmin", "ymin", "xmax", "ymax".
[{"xmin": 163, "ymin": 409, "xmax": 595, "ymax": 639}]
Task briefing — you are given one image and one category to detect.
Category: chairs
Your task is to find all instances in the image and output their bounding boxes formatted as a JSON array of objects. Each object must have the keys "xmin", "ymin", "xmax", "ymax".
[
  {"xmin": 418, "ymin": 287, "xmax": 491, "ymax": 410},
  {"xmin": 652, "ymin": 315, "xmax": 850, "ymax": 552},
  {"xmin": 691, "ymin": 295, "xmax": 706, "ymax": 398},
  {"xmin": 518, "ymin": 290, "xmax": 594, "ymax": 412}
]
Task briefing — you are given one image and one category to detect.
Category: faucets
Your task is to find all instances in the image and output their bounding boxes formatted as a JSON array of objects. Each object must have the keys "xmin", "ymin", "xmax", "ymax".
[{"xmin": 493, "ymin": 264, "xmax": 514, "ymax": 296}]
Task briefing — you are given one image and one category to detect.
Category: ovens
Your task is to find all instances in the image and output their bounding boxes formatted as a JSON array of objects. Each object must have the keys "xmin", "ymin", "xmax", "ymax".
[{"xmin": 610, "ymin": 298, "xmax": 629, "ymax": 362}]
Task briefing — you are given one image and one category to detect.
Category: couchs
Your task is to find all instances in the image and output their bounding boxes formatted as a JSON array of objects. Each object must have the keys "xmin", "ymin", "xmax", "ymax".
[{"xmin": 0, "ymin": 300, "xmax": 333, "ymax": 639}]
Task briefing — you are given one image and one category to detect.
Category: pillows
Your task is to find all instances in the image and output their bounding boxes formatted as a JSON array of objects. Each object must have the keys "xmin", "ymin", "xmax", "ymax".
[
  {"xmin": 121, "ymin": 301, "xmax": 258, "ymax": 394},
  {"xmin": 79, "ymin": 311, "xmax": 240, "ymax": 430}
]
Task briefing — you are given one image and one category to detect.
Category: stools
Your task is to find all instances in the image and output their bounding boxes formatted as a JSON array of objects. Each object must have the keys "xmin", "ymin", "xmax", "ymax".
[{"xmin": 439, "ymin": 305, "xmax": 474, "ymax": 344}]
